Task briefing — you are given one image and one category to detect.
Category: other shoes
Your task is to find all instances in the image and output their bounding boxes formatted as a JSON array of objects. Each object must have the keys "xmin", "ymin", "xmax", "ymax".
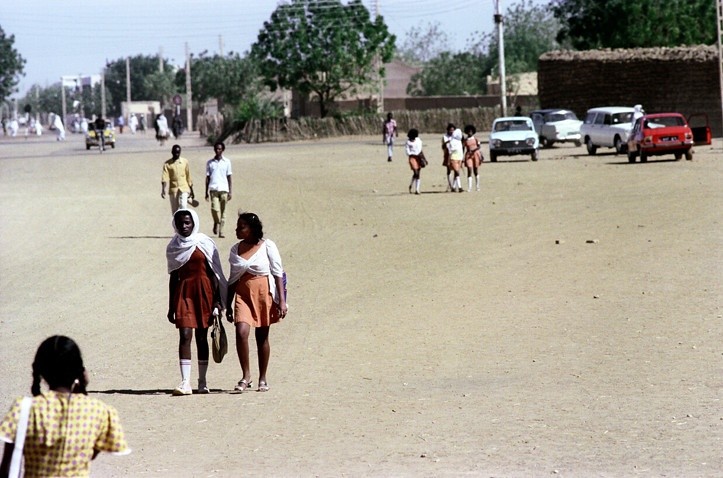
[
  {"xmin": 446, "ymin": 187, "xmax": 480, "ymax": 193},
  {"xmin": 388, "ymin": 156, "xmax": 392, "ymax": 161},
  {"xmin": 214, "ymin": 223, "xmax": 217, "ymax": 233},
  {"xmin": 219, "ymin": 234, "xmax": 225, "ymax": 238},
  {"xmin": 409, "ymin": 185, "xmax": 412, "ymax": 194},
  {"xmin": 416, "ymin": 192, "xmax": 420, "ymax": 194}
]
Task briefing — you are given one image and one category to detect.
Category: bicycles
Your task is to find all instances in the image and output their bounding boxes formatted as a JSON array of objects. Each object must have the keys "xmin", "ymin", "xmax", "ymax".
[{"xmin": 95, "ymin": 130, "xmax": 105, "ymax": 154}]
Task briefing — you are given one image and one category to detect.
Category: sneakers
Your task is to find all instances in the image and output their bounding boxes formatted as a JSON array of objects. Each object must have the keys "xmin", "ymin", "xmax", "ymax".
[
  {"xmin": 198, "ymin": 385, "xmax": 209, "ymax": 393},
  {"xmin": 173, "ymin": 381, "xmax": 193, "ymax": 395}
]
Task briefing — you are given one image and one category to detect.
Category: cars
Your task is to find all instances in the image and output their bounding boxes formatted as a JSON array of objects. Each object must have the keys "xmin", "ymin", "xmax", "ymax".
[
  {"xmin": 489, "ymin": 117, "xmax": 539, "ymax": 162},
  {"xmin": 85, "ymin": 119, "xmax": 116, "ymax": 149},
  {"xmin": 627, "ymin": 112, "xmax": 713, "ymax": 163}
]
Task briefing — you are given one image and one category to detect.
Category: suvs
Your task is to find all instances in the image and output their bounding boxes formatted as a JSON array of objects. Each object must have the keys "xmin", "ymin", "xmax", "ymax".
[
  {"xmin": 530, "ymin": 109, "xmax": 585, "ymax": 149},
  {"xmin": 578, "ymin": 107, "xmax": 665, "ymax": 155}
]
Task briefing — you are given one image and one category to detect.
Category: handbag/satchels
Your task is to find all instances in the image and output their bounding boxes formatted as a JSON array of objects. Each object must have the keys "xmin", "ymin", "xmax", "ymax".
[
  {"xmin": 211, "ymin": 315, "xmax": 228, "ymax": 363},
  {"xmin": 266, "ymin": 240, "xmax": 287, "ymax": 303},
  {"xmin": 417, "ymin": 152, "xmax": 428, "ymax": 168}
]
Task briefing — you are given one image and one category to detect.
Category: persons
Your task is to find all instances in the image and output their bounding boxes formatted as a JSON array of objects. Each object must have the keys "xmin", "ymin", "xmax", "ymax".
[
  {"xmin": 406, "ymin": 129, "xmax": 428, "ymax": 194},
  {"xmin": 383, "ymin": 112, "xmax": 398, "ymax": 161},
  {"xmin": 632, "ymin": 104, "xmax": 644, "ymax": 129},
  {"xmin": 1, "ymin": 108, "xmax": 182, "ymax": 151},
  {"xmin": 205, "ymin": 141, "xmax": 232, "ymax": 238},
  {"xmin": 166, "ymin": 208, "xmax": 288, "ymax": 394},
  {"xmin": 441, "ymin": 123, "xmax": 481, "ymax": 192},
  {"xmin": 0, "ymin": 335, "xmax": 131, "ymax": 478},
  {"xmin": 513, "ymin": 106, "xmax": 523, "ymax": 124},
  {"xmin": 161, "ymin": 145, "xmax": 194, "ymax": 216}
]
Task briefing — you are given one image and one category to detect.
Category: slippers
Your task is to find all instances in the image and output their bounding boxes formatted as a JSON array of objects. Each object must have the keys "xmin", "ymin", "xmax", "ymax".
[
  {"xmin": 234, "ymin": 379, "xmax": 252, "ymax": 393},
  {"xmin": 258, "ymin": 381, "xmax": 269, "ymax": 391}
]
